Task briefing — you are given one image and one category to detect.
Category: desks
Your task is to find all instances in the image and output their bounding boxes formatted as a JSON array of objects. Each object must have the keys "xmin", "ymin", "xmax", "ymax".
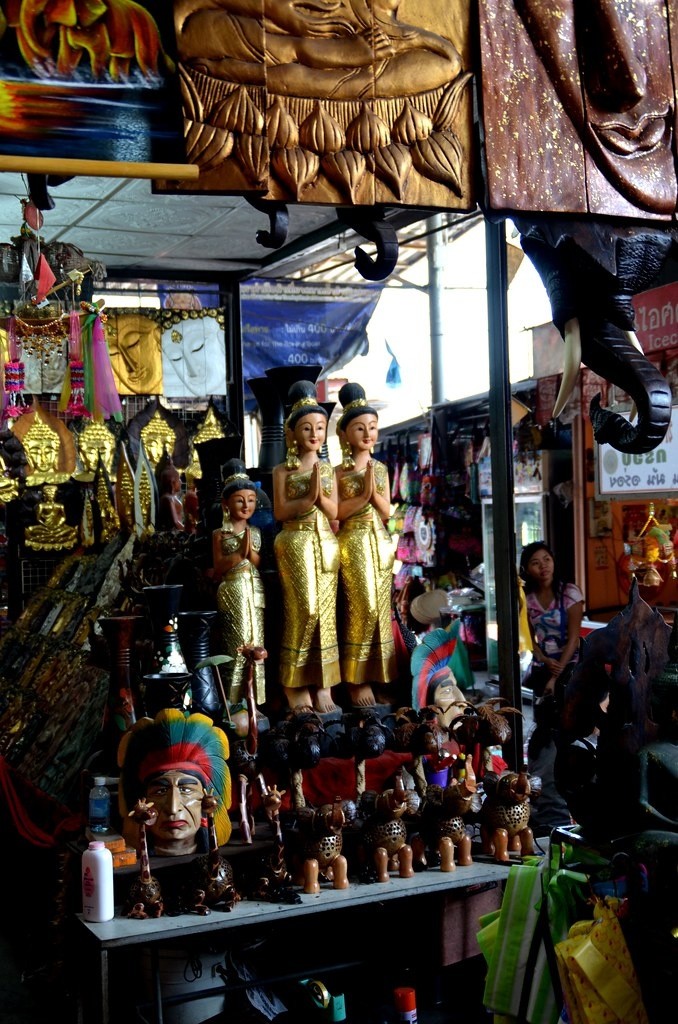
[{"xmin": 74, "ymin": 836, "xmax": 551, "ymax": 1024}]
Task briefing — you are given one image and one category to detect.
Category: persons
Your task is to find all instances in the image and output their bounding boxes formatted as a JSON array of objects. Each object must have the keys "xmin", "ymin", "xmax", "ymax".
[
  {"xmin": 518, "ymin": 541, "xmax": 585, "ymax": 719},
  {"xmin": 631, "ymin": 660, "xmax": 678, "ymax": 862},
  {"xmin": 334, "ymin": 383, "xmax": 399, "ymax": 706},
  {"xmin": 212, "ymin": 459, "xmax": 272, "ymax": 705},
  {"xmin": 116, "ymin": 709, "xmax": 234, "ymax": 855},
  {"xmin": 0, "ymin": 404, "xmax": 241, "ymax": 552},
  {"xmin": 409, "ymin": 628, "xmax": 471, "ymax": 731},
  {"xmin": 273, "ymin": 381, "xmax": 340, "ymax": 715}
]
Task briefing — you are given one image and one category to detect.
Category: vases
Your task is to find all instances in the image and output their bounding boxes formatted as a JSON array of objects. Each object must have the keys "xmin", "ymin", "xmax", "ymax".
[{"xmin": 100, "ymin": 585, "xmax": 222, "ymax": 731}]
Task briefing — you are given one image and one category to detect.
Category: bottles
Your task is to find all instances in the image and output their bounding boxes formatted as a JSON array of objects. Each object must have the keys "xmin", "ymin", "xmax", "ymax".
[
  {"xmin": 90, "ymin": 776, "xmax": 112, "ymax": 835},
  {"xmin": 395, "ymin": 987, "xmax": 418, "ymax": 1024},
  {"xmin": 82, "ymin": 840, "xmax": 115, "ymax": 922}
]
